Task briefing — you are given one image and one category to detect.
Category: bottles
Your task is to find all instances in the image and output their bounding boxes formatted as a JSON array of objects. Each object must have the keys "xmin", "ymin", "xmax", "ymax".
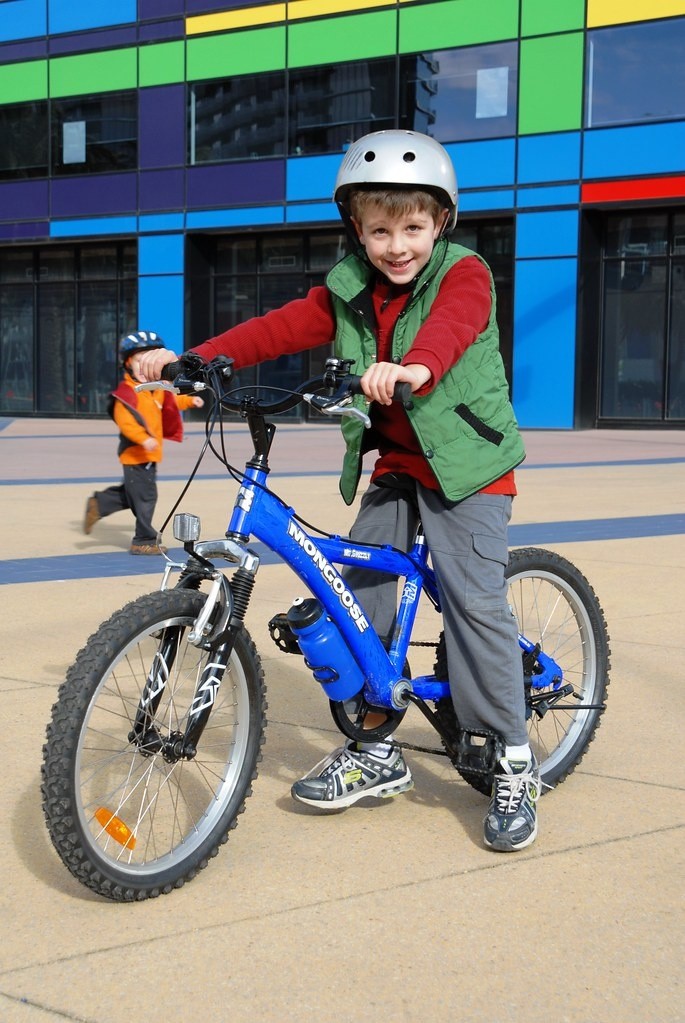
[{"xmin": 286, "ymin": 596, "xmax": 366, "ymax": 702}]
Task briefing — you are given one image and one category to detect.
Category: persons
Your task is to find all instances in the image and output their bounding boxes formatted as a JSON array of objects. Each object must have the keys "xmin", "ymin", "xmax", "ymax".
[
  {"xmin": 138, "ymin": 130, "xmax": 540, "ymax": 851},
  {"xmin": 84, "ymin": 331, "xmax": 204, "ymax": 555}
]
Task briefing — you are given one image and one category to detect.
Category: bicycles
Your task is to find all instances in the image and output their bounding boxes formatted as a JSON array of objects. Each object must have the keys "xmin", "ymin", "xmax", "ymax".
[{"xmin": 38, "ymin": 349, "xmax": 613, "ymax": 905}]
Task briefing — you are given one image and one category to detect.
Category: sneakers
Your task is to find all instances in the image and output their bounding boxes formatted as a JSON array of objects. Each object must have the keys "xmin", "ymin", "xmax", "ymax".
[
  {"xmin": 484, "ymin": 739, "xmax": 555, "ymax": 851},
  {"xmin": 130, "ymin": 543, "xmax": 168, "ymax": 556},
  {"xmin": 83, "ymin": 497, "xmax": 101, "ymax": 534},
  {"xmin": 290, "ymin": 735, "xmax": 414, "ymax": 809}
]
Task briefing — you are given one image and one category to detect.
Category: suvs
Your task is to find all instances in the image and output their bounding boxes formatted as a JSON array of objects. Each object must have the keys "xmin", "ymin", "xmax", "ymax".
[
  {"xmin": 1, "ymin": 359, "xmax": 34, "ymax": 410},
  {"xmin": 614, "ymin": 355, "xmax": 673, "ymax": 420}
]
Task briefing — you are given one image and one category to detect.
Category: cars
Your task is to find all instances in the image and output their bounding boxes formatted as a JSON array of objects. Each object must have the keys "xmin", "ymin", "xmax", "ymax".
[{"xmin": 67, "ymin": 360, "xmax": 118, "ymax": 412}]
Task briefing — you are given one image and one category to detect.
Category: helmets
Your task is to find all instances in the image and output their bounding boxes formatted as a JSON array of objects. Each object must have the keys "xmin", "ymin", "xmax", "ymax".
[
  {"xmin": 332, "ymin": 129, "xmax": 459, "ymax": 232},
  {"xmin": 117, "ymin": 331, "xmax": 164, "ymax": 355}
]
